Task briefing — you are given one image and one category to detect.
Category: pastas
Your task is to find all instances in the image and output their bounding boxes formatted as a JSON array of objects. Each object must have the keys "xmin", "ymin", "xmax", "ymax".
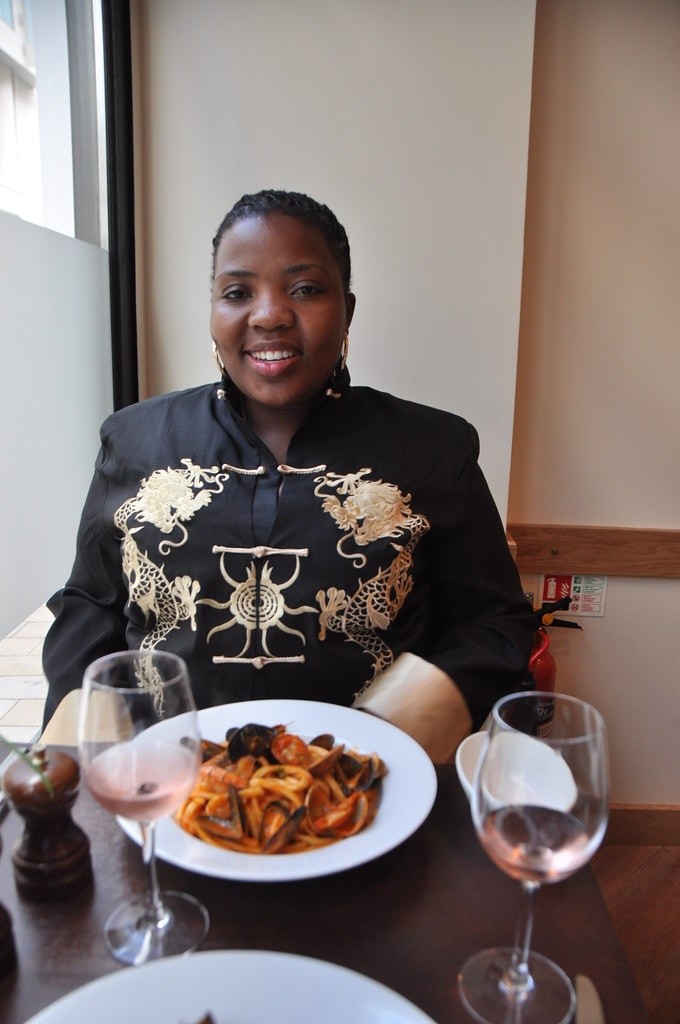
[{"xmin": 175, "ymin": 744, "xmax": 377, "ymax": 855}]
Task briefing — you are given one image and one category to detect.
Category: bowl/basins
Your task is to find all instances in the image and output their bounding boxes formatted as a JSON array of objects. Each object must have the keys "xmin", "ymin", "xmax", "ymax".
[{"xmin": 455, "ymin": 731, "xmax": 578, "ymax": 816}]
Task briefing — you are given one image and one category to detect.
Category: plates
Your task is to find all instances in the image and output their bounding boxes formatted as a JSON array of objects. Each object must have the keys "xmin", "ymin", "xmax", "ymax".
[
  {"xmin": 23, "ymin": 950, "xmax": 437, "ymax": 1023},
  {"xmin": 114, "ymin": 700, "xmax": 438, "ymax": 882}
]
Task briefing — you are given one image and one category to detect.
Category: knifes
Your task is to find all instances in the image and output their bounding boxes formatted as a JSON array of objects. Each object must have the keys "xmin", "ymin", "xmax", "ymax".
[{"xmin": 576, "ymin": 974, "xmax": 604, "ymax": 1024}]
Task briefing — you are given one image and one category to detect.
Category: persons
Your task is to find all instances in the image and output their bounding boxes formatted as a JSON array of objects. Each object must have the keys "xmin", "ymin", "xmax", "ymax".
[{"xmin": 36, "ymin": 190, "xmax": 535, "ymax": 767}]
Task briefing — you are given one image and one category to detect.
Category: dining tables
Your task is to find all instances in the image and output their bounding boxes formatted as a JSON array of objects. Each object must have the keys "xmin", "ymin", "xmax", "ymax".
[{"xmin": 0, "ymin": 744, "xmax": 651, "ymax": 1024}]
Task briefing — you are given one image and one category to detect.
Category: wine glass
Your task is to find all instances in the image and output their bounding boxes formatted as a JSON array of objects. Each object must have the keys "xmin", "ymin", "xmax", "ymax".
[
  {"xmin": 457, "ymin": 691, "xmax": 608, "ymax": 1024},
  {"xmin": 78, "ymin": 650, "xmax": 210, "ymax": 965}
]
